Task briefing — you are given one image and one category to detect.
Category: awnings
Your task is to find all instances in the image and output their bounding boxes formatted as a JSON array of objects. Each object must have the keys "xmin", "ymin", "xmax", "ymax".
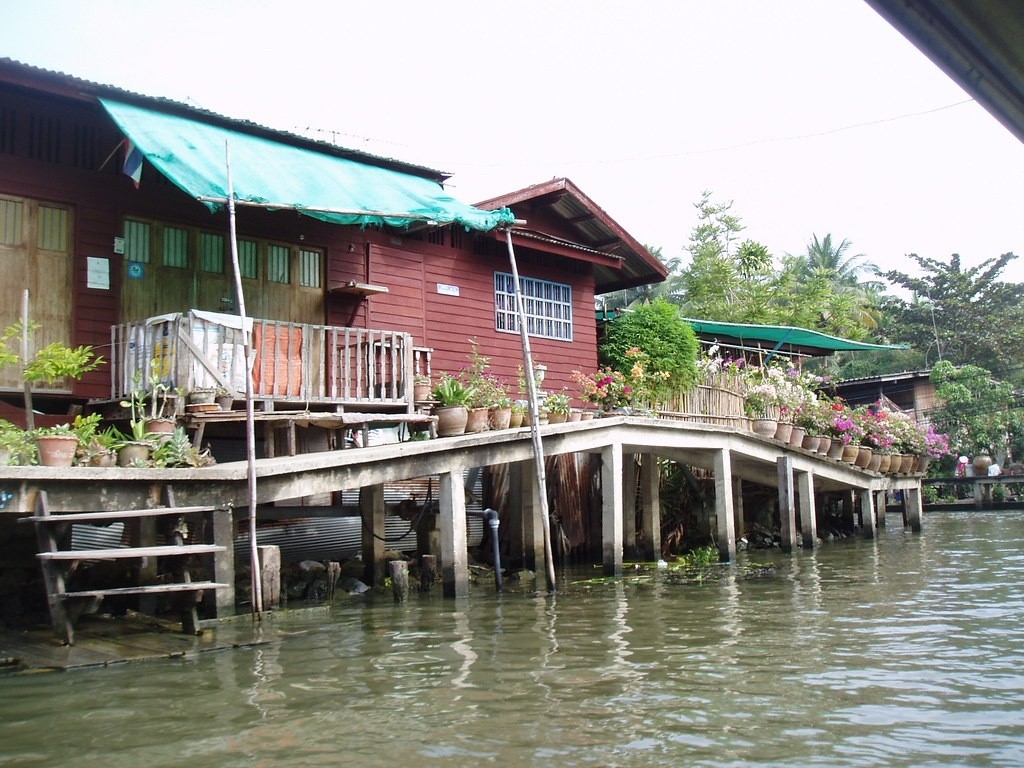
[
  {"xmin": 595, "ymin": 303, "xmax": 909, "ymax": 366},
  {"xmin": 101, "ymin": 96, "xmax": 557, "ymax": 617}
]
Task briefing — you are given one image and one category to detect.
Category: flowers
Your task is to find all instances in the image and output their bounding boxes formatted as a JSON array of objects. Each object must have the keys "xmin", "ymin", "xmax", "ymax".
[
  {"xmin": 542, "ymin": 384, "xmax": 573, "ymax": 414},
  {"xmin": 448, "ymin": 366, "xmax": 515, "ymax": 408},
  {"xmin": 694, "ymin": 342, "xmax": 965, "ymax": 479},
  {"xmin": 569, "ymin": 347, "xmax": 670, "ymax": 414}
]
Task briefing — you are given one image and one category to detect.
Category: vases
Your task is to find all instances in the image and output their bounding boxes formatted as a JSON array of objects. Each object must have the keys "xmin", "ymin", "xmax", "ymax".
[
  {"xmin": 790, "ymin": 426, "xmax": 805, "ymax": 448},
  {"xmin": 867, "ymin": 452, "xmax": 882, "ymax": 472},
  {"xmin": 842, "ymin": 446, "xmax": 860, "ymax": 464},
  {"xmin": 582, "ymin": 412, "xmax": 594, "ymax": 420},
  {"xmin": 489, "ymin": 406, "xmax": 512, "ymax": 430},
  {"xmin": 750, "ymin": 418, "xmax": 777, "ymax": 439},
  {"xmin": 855, "ymin": 446, "xmax": 873, "ymax": 469},
  {"xmin": 890, "ymin": 454, "xmax": 902, "ymax": 473},
  {"xmin": 802, "ymin": 435, "xmax": 820, "ymax": 453},
  {"xmin": 775, "ymin": 422, "xmax": 795, "ymax": 443},
  {"xmin": 898, "ymin": 454, "xmax": 915, "ymax": 473},
  {"xmin": 828, "ymin": 441, "xmax": 845, "ymax": 460},
  {"xmin": 878, "ymin": 453, "xmax": 891, "ymax": 473},
  {"xmin": 816, "ymin": 435, "xmax": 832, "ymax": 456},
  {"xmin": 569, "ymin": 411, "xmax": 582, "ymax": 422},
  {"xmin": 917, "ymin": 456, "xmax": 929, "ymax": 473},
  {"xmin": 548, "ymin": 412, "xmax": 567, "ymax": 423},
  {"xmin": 911, "ymin": 455, "xmax": 921, "ymax": 473}
]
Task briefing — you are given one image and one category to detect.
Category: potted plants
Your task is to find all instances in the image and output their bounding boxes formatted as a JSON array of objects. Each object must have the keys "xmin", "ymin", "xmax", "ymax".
[
  {"xmin": 0, "ymin": 358, "xmax": 234, "ymax": 468},
  {"xmin": 413, "ymin": 336, "xmax": 548, "ymax": 438}
]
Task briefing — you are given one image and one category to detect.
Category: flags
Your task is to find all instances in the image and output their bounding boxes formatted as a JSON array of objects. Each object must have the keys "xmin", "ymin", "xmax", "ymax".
[{"xmin": 122, "ymin": 139, "xmax": 143, "ymax": 190}]
